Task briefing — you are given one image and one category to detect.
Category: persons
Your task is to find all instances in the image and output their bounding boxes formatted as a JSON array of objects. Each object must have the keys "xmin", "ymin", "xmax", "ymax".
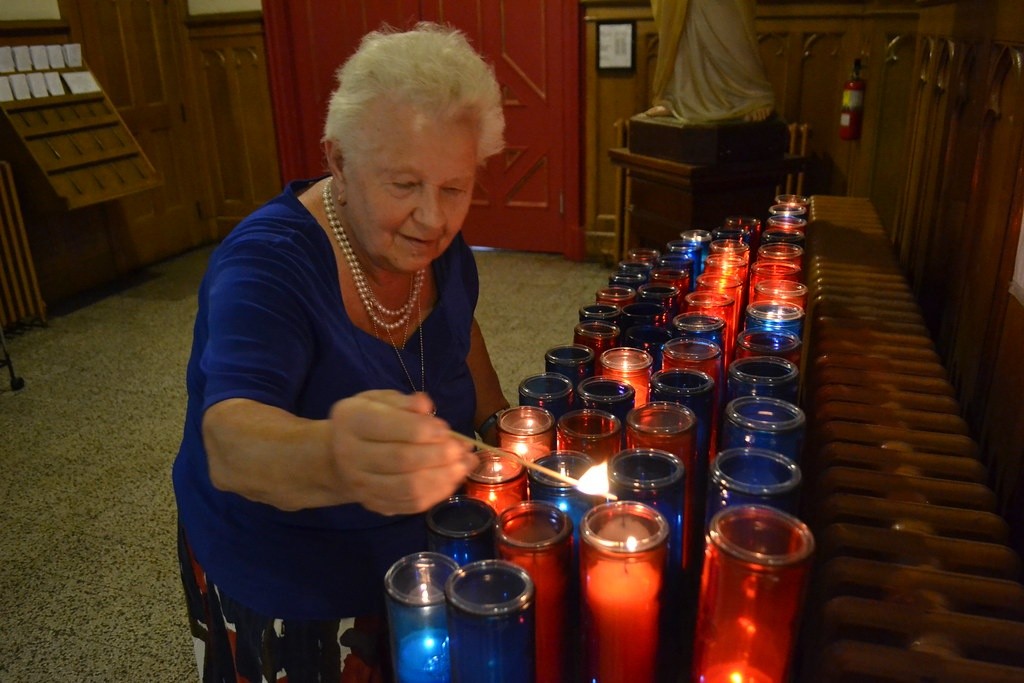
[{"xmin": 171, "ymin": 30, "xmax": 508, "ymax": 683}]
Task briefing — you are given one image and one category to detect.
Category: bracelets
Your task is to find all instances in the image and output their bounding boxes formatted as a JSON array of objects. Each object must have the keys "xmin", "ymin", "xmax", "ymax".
[{"xmin": 478, "ymin": 406, "xmax": 508, "ymax": 437}]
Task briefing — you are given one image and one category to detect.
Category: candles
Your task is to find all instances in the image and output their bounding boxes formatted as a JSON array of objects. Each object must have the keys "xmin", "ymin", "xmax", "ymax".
[{"xmin": 383, "ymin": 197, "xmax": 866, "ymax": 683}]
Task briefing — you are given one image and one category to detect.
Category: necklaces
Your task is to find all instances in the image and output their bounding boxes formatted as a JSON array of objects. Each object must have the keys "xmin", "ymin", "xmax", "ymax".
[{"xmin": 323, "ymin": 176, "xmax": 437, "ymax": 416}]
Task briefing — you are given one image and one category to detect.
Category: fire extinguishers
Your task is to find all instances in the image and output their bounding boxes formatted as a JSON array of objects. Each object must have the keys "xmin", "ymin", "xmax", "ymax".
[{"xmin": 837, "ymin": 57, "xmax": 866, "ymax": 142}]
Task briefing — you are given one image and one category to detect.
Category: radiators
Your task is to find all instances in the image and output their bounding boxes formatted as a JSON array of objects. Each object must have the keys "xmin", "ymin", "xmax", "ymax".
[{"xmin": 804, "ymin": 192, "xmax": 1024, "ymax": 683}]
